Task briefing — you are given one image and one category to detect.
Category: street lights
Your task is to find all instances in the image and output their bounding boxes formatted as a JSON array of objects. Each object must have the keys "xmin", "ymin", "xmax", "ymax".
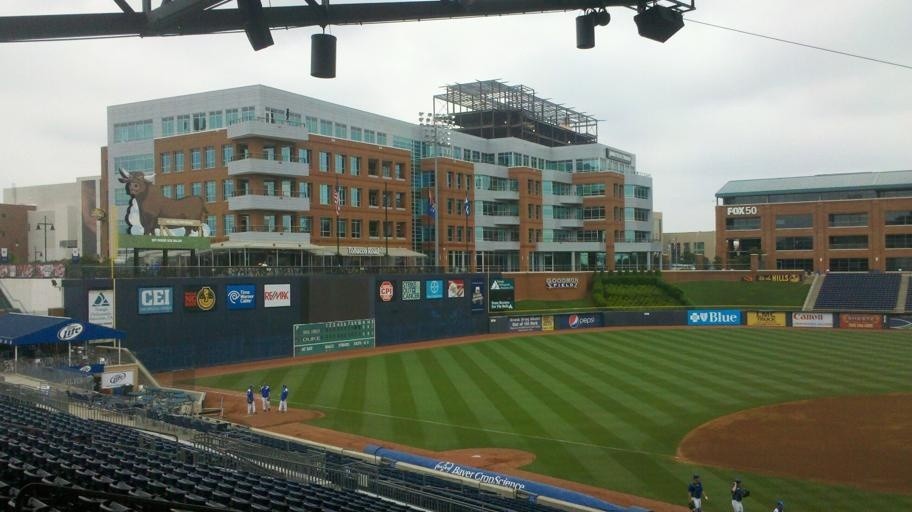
[
  {"xmin": 32, "ymin": 245, "xmax": 43, "ymax": 278},
  {"xmin": 672, "ymin": 238, "xmax": 679, "ymax": 269},
  {"xmin": 35, "ymin": 215, "xmax": 56, "ymax": 263},
  {"xmin": 14, "ymin": 238, "xmax": 29, "ymax": 279},
  {"xmin": 202, "ymin": 237, "xmax": 308, "ymax": 275}
]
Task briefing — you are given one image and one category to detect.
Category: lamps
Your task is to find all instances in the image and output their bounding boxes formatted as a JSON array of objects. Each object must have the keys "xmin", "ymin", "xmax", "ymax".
[
  {"xmin": 311, "ymin": 28, "xmax": 337, "ymax": 79},
  {"xmin": 634, "ymin": 3, "xmax": 686, "ymax": 43},
  {"xmin": 577, "ymin": 9, "xmax": 594, "ymax": 49}
]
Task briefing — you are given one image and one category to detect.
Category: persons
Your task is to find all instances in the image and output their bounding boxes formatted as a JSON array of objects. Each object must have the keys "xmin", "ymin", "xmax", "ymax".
[
  {"xmin": 687, "ymin": 474, "xmax": 709, "ymax": 512},
  {"xmin": 729, "ymin": 478, "xmax": 750, "ymax": 512},
  {"xmin": 246, "ymin": 384, "xmax": 258, "ymax": 416},
  {"xmin": 277, "ymin": 384, "xmax": 290, "ymax": 413},
  {"xmin": 772, "ymin": 500, "xmax": 784, "ymax": 512},
  {"xmin": 232, "ymin": 261, "xmax": 303, "ymax": 279},
  {"xmin": 259, "ymin": 383, "xmax": 273, "ymax": 413}
]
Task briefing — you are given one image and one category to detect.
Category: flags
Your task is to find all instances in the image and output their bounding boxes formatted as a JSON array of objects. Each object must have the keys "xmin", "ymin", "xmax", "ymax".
[
  {"xmin": 426, "ymin": 187, "xmax": 437, "ymax": 220},
  {"xmin": 464, "ymin": 196, "xmax": 471, "ymax": 217},
  {"xmin": 333, "ymin": 179, "xmax": 341, "ymax": 217}
]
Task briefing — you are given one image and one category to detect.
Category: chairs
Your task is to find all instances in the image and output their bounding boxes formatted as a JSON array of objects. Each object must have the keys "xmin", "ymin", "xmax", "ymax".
[{"xmin": 0, "ymin": 393, "xmax": 567, "ymax": 512}]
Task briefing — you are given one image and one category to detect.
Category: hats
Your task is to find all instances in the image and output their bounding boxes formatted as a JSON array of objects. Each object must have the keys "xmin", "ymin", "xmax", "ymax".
[
  {"xmin": 694, "ymin": 475, "xmax": 700, "ymax": 479},
  {"xmin": 736, "ymin": 479, "xmax": 742, "ymax": 483},
  {"xmin": 778, "ymin": 500, "xmax": 784, "ymax": 506}
]
